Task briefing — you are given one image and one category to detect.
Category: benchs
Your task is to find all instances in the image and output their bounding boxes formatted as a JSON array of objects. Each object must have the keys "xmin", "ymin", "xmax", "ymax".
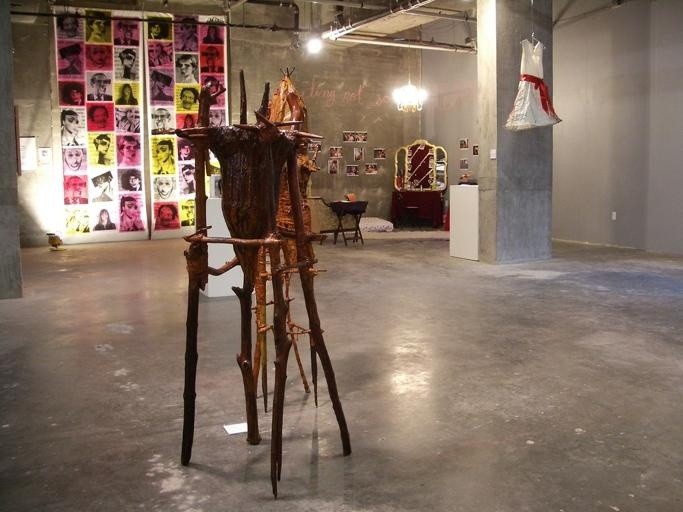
[{"xmin": 277, "ymin": 197, "xmax": 361, "ymax": 245}]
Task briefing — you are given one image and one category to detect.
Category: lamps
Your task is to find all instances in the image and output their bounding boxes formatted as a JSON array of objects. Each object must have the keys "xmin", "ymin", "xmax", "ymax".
[{"xmin": 395, "ymin": 43, "xmax": 425, "ymax": 112}]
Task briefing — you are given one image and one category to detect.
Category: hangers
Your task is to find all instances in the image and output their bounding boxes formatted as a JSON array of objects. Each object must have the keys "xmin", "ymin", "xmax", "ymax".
[{"xmin": 520, "ymin": 32, "xmax": 546, "ymax": 49}]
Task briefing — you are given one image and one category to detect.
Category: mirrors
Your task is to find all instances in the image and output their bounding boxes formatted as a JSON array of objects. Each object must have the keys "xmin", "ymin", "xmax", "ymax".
[{"xmin": 395, "ymin": 138, "xmax": 449, "ymax": 193}]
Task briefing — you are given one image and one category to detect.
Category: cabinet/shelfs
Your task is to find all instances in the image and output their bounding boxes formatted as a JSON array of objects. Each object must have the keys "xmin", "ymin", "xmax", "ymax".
[{"xmin": 392, "ymin": 185, "xmax": 480, "ymax": 260}]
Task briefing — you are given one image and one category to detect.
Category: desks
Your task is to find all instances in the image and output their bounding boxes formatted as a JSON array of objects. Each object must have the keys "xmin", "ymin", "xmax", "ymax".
[{"xmin": 332, "ymin": 199, "xmax": 368, "ymax": 246}]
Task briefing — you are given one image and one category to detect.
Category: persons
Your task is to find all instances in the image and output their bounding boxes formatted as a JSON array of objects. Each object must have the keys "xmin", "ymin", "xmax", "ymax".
[
  {"xmin": 55, "ymin": 10, "xmax": 224, "ymax": 235},
  {"xmin": 307, "ymin": 133, "xmax": 385, "ymax": 176}
]
